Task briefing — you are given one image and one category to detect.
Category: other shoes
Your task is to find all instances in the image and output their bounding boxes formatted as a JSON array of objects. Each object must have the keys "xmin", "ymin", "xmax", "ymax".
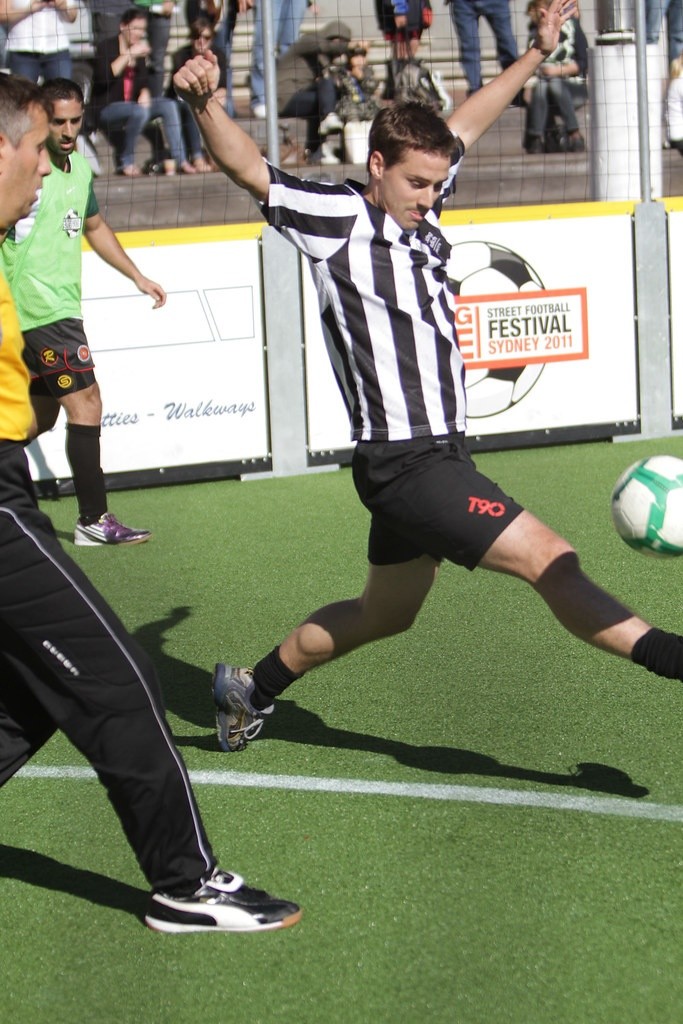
[
  {"xmin": 568, "ymin": 133, "xmax": 586, "ymax": 153},
  {"xmin": 124, "ymin": 166, "xmax": 144, "ymax": 177},
  {"xmin": 317, "ymin": 112, "xmax": 344, "ymax": 135},
  {"xmin": 179, "ymin": 160, "xmax": 196, "ymax": 174},
  {"xmin": 524, "ymin": 136, "xmax": 542, "ymax": 154},
  {"xmin": 309, "ymin": 144, "xmax": 340, "ymax": 165},
  {"xmin": 193, "ymin": 157, "xmax": 212, "ymax": 173},
  {"xmin": 253, "ymin": 104, "xmax": 268, "ymax": 120}
]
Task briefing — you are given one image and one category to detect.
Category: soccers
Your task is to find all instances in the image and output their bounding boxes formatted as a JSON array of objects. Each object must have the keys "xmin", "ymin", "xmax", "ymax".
[{"xmin": 609, "ymin": 454, "xmax": 683, "ymax": 561}]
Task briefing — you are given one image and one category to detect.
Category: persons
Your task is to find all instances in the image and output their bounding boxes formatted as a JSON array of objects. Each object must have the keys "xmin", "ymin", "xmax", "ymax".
[
  {"xmin": 3, "ymin": 81, "xmax": 166, "ymax": 545},
  {"xmin": 526, "ymin": 0, "xmax": 590, "ymax": 153},
  {"xmin": 0, "ymin": 78, "xmax": 304, "ymax": 932},
  {"xmin": 174, "ymin": 0, "xmax": 683, "ymax": 751},
  {"xmin": 647, "ymin": 0, "xmax": 683, "ymax": 154},
  {"xmin": 449, "ymin": 0, "xmax": 524, "ymax": 108},
  {"xmin": 374, "ymin": 0, "xmax": 433, "ymax": 99},
  {"xmin": 279, "ymin": 20, "xmax": 380, "ymax": 166},
  {"xmin": 1, "ymin": 0, "xmax": 319, "ymax": 176}
]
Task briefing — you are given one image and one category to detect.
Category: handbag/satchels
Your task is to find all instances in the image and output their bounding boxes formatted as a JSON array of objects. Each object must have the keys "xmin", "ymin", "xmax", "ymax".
[{"xmin": 381, "ymin": 30, "xmax": 443, "ymax": 113}]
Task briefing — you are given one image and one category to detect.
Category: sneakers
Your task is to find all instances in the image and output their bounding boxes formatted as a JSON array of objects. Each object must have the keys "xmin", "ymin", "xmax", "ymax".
[
  {"xmin": 73, "ymin": 512, "xmax": 151, "ymax": 547},
  {"xmin": 145, "ymin": 866, "xmax": 305, "ymax": 934},
  {"xmin": 212, "ymin": 664, "xmax": 276, "ymax": 754}
]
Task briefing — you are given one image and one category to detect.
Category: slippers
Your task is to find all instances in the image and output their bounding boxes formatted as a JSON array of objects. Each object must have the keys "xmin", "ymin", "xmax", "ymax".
[{"xmin": 196, "ymin": 34, "xmax": 212, "ymax": 40}]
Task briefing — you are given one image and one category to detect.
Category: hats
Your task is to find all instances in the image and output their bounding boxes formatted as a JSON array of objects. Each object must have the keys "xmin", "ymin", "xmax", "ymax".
[{"xmin": 322, "ymin": 21, "xmax": 352, "ymax": 42}]
